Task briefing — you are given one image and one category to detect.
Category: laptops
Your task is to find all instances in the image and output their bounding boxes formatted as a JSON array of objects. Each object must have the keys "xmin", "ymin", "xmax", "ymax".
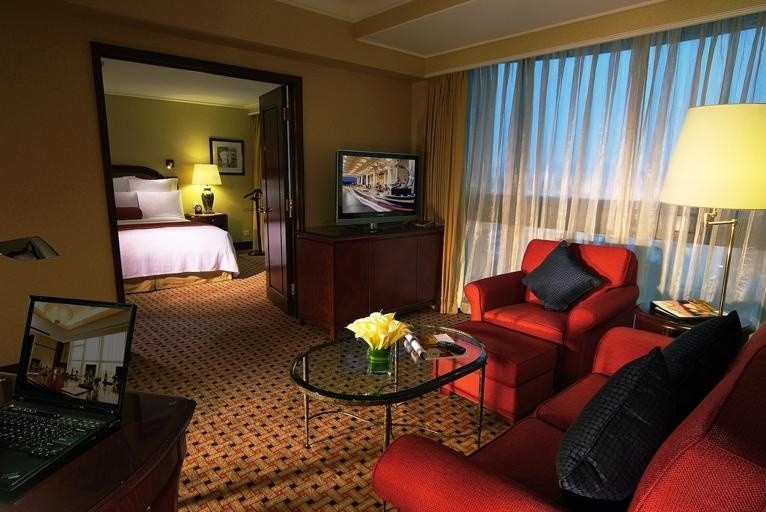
[{"xmin": 1, "ymin": 296, "xmax": 137, "ymax": 504}]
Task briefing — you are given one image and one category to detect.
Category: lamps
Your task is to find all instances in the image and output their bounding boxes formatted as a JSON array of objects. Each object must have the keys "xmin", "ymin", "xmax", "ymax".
[
  {"xmin": 191, "ymin": 164, "xmax": 222, "ymax": 213},
  {"xmin": 658, "ymin": 104, "xmax": 766, "ymax": 315}
]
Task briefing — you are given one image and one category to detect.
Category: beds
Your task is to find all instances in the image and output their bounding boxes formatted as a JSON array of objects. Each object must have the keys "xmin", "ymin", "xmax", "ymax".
[{"xmin": 111, "ymin": 165, "xmax": 240, "ymax": 295}]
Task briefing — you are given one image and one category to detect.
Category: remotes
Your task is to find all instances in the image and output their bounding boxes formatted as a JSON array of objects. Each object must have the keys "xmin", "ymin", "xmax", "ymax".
[{"xmin": 437, "ymin": 341, "xmax": 466, "ymax": 354}]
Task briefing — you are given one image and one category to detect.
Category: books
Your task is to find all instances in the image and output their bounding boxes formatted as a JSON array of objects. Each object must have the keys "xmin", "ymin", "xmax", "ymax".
[
  {"xmin": 649, "ymin": 299, "xmax": 729, "ymax": 327},
  {"xmin": 405, "ymin": 333, "xmax": 468, "ymax": 360}
]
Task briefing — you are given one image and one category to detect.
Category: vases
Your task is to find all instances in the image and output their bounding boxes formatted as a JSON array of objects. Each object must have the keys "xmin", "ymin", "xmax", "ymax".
[{"xmin": 367, "ymin": 349, "xmax": 393, "ymax": 375}]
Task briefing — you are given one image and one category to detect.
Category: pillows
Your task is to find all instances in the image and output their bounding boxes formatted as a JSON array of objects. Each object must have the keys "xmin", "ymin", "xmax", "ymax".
[
  {"xmin": 113, "ymin": 176, "xmax": 185, "ymax": 222},
  {"xmin": 521, "ymin": 240, "xmax": 603, "ymax": 311},
  {"xmin": 662, "ymin": 310, "xmax": 742, "ymax": 423},
  {"xmin": 554, "ymin": 345, "xmax": 674, "ymax": 501}
]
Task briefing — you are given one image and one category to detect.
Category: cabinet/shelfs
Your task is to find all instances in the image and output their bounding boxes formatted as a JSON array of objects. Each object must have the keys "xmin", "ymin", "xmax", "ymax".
[{"xmin": 296, "ymin": 220, "xmax": 444, "ymax": 341}]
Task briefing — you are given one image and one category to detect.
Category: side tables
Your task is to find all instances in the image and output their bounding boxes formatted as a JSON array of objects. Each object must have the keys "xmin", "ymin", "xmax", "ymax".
[{"xmin": 634, "ymin": 303, "xmax": 755, "ymax": 343}]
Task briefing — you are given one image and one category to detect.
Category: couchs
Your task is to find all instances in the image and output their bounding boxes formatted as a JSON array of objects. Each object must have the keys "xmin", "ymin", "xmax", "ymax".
[
  {"xmin": 432, "ymin": 239, "xmax": 639, "ymax": 424},
  {"xmin": 372, "ymin": 322, "xmax": 766, "ymax": 512}
]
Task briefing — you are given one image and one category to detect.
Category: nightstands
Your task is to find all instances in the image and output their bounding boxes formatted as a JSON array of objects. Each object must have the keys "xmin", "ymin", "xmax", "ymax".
[{"xmin": 185, "ymin": 212, "xmax": 227, "ymax": 232}]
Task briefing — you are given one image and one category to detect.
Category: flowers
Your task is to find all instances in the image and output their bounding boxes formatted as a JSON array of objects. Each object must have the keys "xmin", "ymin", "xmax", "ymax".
[{"xmin": 344, "ymin": 309, "xmax": 415, "ymax": 349}]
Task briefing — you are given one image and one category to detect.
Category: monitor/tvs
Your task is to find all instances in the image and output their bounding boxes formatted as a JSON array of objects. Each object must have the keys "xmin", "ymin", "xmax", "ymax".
[{"xmin": 335, "ymin": 148, "xmax": 419, "ymax": 233}]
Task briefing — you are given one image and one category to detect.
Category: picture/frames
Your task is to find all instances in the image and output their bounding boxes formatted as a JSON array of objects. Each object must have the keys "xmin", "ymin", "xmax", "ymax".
[{"xmin": 209, "ymin": 137, "xmax": 245, "ymax": 175}]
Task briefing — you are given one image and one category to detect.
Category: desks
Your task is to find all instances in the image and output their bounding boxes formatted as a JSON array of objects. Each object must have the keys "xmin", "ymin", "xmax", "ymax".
[{"xmin": 1, "ymin": 373, "xmax": 196, "ymax": 512}]
